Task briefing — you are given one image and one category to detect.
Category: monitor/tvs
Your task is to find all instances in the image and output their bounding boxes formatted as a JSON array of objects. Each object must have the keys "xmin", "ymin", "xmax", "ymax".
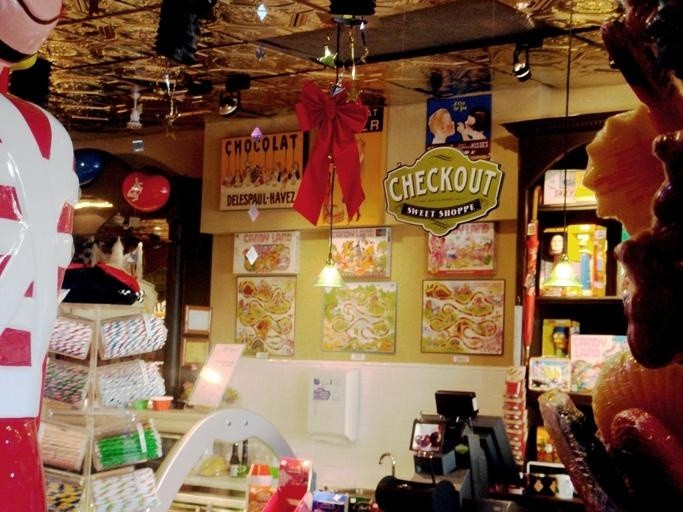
[{"xmin": 419, "ymin": 411, "xmax": 520, "ymax": 487}]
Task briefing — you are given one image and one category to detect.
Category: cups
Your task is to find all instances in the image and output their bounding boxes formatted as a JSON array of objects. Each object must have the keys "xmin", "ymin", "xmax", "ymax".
[{"xmin": 132, "ymin": 395, "xmax": 173, "ymax": 411}]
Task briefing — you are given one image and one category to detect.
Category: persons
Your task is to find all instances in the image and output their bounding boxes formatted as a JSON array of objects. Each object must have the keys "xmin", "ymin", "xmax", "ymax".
[{"xmin": 428, "ymin": 108, "xmax": 491, "ymax": 145}]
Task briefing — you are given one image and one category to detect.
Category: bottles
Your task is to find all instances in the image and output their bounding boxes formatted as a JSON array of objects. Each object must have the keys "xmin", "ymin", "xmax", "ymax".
[
  {"xmin": 229, "ymin": 439, "xmax": 249, "ymax": 478},
  {"xmin": 246, "ymin": 461, "xmax": 273, "ymax": 512}
]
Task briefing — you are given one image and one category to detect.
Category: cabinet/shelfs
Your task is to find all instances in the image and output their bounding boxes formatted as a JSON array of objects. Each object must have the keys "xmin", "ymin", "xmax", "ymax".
[{"xmin": 499, "ymin": 107, "xmax": 630, "ymax": 512}]
